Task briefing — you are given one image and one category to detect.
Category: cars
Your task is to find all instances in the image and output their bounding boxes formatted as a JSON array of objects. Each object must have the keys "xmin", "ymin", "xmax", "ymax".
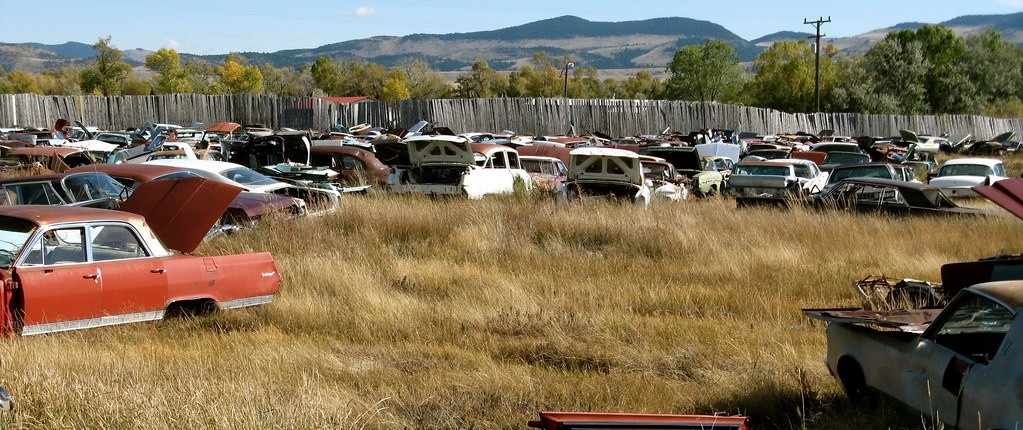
[
  {"xmin": 0, "ymin": 117, "xmax": 1023, "ymax": 234},
  {"xmin": 0, "ymin": 177, "xmax": 282, "ymax": 336},
  {"xmin": 824, "ymin": 279, "xmax": 1023, "ymax": 430}
]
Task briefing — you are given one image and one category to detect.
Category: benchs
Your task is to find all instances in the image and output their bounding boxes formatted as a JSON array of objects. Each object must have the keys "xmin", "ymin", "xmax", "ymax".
[{"xmin": 48, "ymin": 247, "xmax": 137, "ymax": 266}]
